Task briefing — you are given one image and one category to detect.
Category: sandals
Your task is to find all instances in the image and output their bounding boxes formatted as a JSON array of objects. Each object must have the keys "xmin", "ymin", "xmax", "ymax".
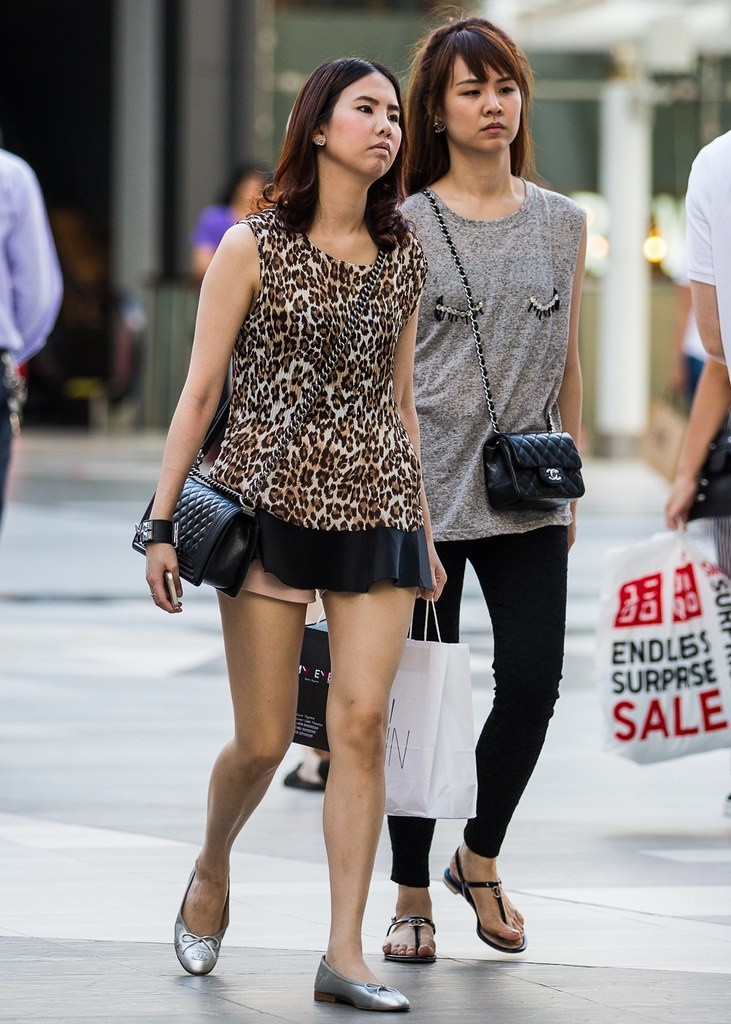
[
  {"xmin": 381, "ymin": 913, "xmax": 437, "ymax": 963},
  {"xmin": 441, "ymin": 845, "xmax": 527, "ymax": 954}
]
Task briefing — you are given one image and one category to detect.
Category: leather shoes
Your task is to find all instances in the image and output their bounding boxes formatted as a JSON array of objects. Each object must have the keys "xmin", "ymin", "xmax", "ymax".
[
  {"xmin": 173, "ymin": 865, "xmax": 229, "ymax": 975},
  {"xmin": 313, "ymin": 954, "xmax": 411, "ymax": 1012}
]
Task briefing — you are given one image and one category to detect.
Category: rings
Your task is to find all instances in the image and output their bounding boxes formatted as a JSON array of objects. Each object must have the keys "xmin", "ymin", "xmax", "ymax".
[{"xmin": 150, "ymin": 593, "xmax": 155, "ymax": 596}]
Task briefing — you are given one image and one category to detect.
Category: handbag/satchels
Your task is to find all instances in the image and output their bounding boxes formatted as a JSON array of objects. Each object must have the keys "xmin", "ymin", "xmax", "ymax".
[
  {"xmin": 595, "ymin": 517, "xmax": 731, "ymax": 765},
  {"xmin": 290, "ymin": 610, "xmax": 330, "ymax": 752},
  {"xmin": 688, "ymin": 433, "xmax": 731, "ymax": 520},
  {"xmin": 131, "ymin": 465, "xmax": 261, "ymax": 599},
  {"xmin": 381, "ymin": 595, "xmax": 479, "ymax": 821},
  {"xmin": 482, "ymin": 431, "xmax": 585, "ymax": 511}
]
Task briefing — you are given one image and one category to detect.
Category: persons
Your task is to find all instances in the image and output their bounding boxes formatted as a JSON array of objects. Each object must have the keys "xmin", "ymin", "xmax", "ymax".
[
  {"xmin": 664, "ymin": 127, "xmax": 731, "ymax": 815},
  {"xmin": 382, "ymin": 19, "xmax": 588, "ymax": 964},
  {"xmin": 188, "ymin": 163, "xmax": 274, "ymax": 283},
  {"xmin": 135, "ymin": 58, "xmax": 448, "ymax": 1010},
  {"xmin": 0, "ymin": 149, "xmax": 61, "ymax": 532}
]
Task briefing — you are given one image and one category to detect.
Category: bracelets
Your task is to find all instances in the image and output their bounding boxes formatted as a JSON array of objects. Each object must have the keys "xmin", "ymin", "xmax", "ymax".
[{"xmin": 135, "ymin": 519, "xmax": 181, "ymax": 548}]
